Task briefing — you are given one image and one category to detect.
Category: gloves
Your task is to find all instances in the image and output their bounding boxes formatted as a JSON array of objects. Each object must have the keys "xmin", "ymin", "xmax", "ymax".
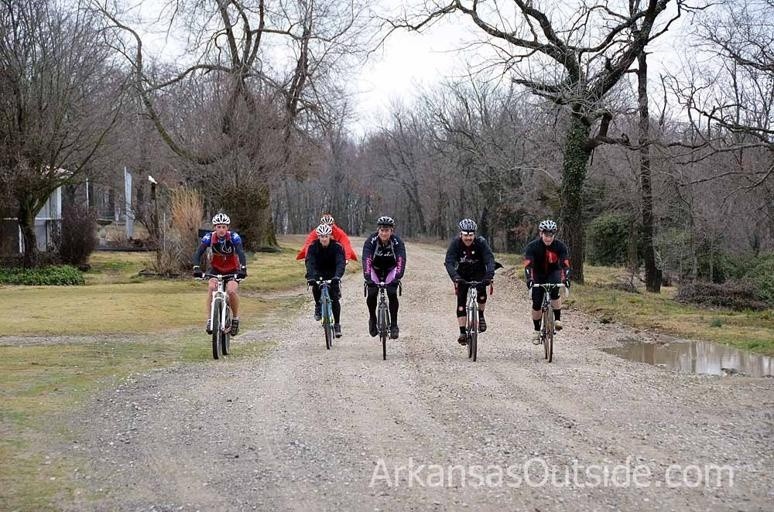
[
  {"xmin": 309, "ymin": 278, "xmax": 319, "ymax": 285},
  {"xmin": 391, "ymin": 276, "xmax": 401, "ymax": 286},
  {"xmin": 565, "ymin": 277, "xmax": 571, "ymax": 287},
  {"xmin": 367, "ymin": 277, "xmax": 376, "ymax": 288},
  {"xmin": 193, "ymin": 267, "xmax": 204, "ymax": 277},
  {"xmin": 456, "ymin": 279, "xmax": 467, "ymax": 285},
  {"xmin": 237, "ymin": 268, "xmax": 246, "ymax": 278},
  {"xmin": 332, "ymin": 276, "xmax": 340, "ymax": 284},
  {"xmin": 526, "ymin": 277, "xmax": 535, "ymax": 289},
  {"xmin": 483, "ymin": 279, "xmax": 491, "ymax": 285}
]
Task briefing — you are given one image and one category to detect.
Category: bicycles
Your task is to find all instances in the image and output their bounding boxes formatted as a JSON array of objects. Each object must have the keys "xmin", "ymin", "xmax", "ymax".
[
  {"xmin": 194, "ymin": 269, "xmax": 248, "ymax": 360},
  {"xmin": 362, "ymin": 280, "xmax": 403, "ymax": 360},
  {"xmin": 306, "ymin": 277, "xmax": 342, "ymax": 349},
  {"xmin": 528, "ymin": 279, "xmax": 569, "ymax": 364},
  {"xmin": 453, "ymin": 277, "xmax": 493, "ymax": 362}
]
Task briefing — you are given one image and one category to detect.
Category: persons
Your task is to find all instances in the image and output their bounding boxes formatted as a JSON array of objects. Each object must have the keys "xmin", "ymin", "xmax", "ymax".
[
  {"xmin": 303, "ymin": 215, "xmax": 351, "ymax": 267},
  {"xmin": 523, "ymin": 219, "xmax": 571, "ymax": 345},
  {"xmin": 360, "ymin": 216, "xmax": 407, "ymax": 340},
  {"xmin": 305, "ymin": 225, "xmax": 345, "ymax": 339},
  {"xmin": 442, "ymin": 217, "xmax": 503, "ymax": 346},
  {"xmin": 191, "ymin": 213, "xmax": 246, "ymax": 335}
]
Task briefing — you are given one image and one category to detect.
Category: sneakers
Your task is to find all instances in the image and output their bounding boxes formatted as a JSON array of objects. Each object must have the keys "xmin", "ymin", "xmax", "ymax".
[
  {"xmin": 389, "ymin": 324, "xmax": 398, "ymax": 339},
  {"xmin": 334, "ymin": 324, "xmax": 341, "ymax": 337},
  {"xmin": 230, "ymin": 319, "xmax": 240, "ymax": 335},
  {"xmin": 368, "ymin": 318, "xmax": 378, "ymax": 336},
  {"xmin": 556, "ymin": 320, "xmax": 563, "ymax": 331},
  {"xmin": 207, "ymin": 320, "xmax": 212, "ymax": 335},
  {"xmin": 479, "ymin": 315, "xmax": 487, "ymax": 332},
  {"xmin": 532, "ymin": 329, "xmax": 541, "ymax": 346},
  {"xmin": 314, "ymin": 304, "xmax": 323, "ymax": 320},
  {"xmin": 456, "ymin": 334, "xmax": 467, "ymax": 342}
]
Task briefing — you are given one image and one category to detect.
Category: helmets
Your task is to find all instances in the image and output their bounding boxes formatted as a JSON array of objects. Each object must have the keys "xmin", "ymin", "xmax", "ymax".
[
  {"xmin": 316, "ymin": 223, "xmax": 333, "ymax": 235},
  {"xmin": 321, "ymin": 215, "xmax": 336, "ymax": 225},
  {"xmin": 211, "ymin": 213, "xmax": 231, "ymax": 226},
  {"xmin": 377, "ymin": 216, "xmax": 396, "ymax": 226}
]
